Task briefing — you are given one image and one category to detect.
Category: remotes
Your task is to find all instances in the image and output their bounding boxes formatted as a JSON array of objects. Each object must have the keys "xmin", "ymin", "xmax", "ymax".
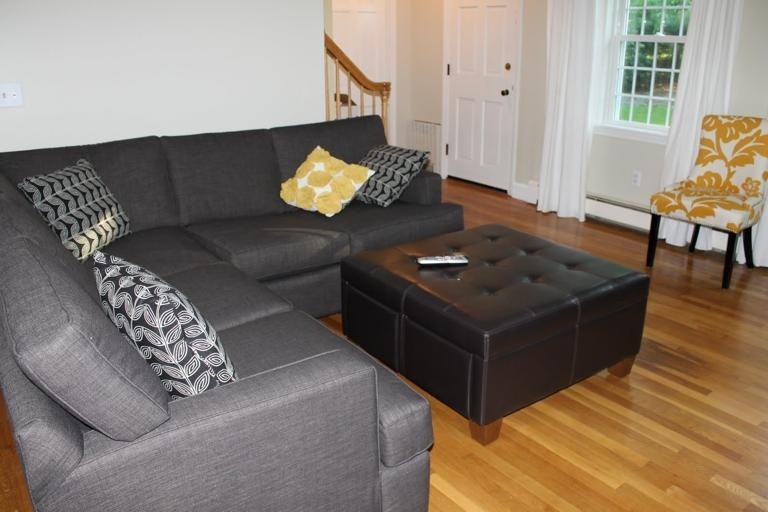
[{"xmin": 416, "ymin": 255, "xmax": 470, "ymax": 266}]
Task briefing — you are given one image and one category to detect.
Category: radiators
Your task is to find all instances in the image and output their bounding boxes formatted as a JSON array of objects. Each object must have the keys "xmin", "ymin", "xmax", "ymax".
[{"xmin": 413, "ymin": 119, "xmax": 441, "ymax": 167}]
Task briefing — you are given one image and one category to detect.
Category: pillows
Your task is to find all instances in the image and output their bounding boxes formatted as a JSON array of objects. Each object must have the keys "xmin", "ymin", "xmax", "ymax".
[{"xmin": 277, "ymin": 140, "xmax": 432, "ymax": 218}]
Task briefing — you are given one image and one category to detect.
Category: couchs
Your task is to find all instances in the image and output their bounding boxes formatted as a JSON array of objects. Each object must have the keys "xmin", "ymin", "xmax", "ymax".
[{"xmin": 0, "ymin": 113, "xmax": 464, "ymax": 510}]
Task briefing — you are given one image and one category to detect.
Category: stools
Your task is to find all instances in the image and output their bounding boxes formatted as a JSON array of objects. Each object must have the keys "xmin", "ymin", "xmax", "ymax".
[{"xmin": 338, "ymin": 223, "xmax": 649, "ymax": 446}]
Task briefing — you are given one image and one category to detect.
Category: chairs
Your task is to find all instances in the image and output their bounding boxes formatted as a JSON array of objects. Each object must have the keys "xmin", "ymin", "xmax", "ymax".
[{"xmin": 645, "ymin": 113, "xmax": 768, "ymax": 289}]
